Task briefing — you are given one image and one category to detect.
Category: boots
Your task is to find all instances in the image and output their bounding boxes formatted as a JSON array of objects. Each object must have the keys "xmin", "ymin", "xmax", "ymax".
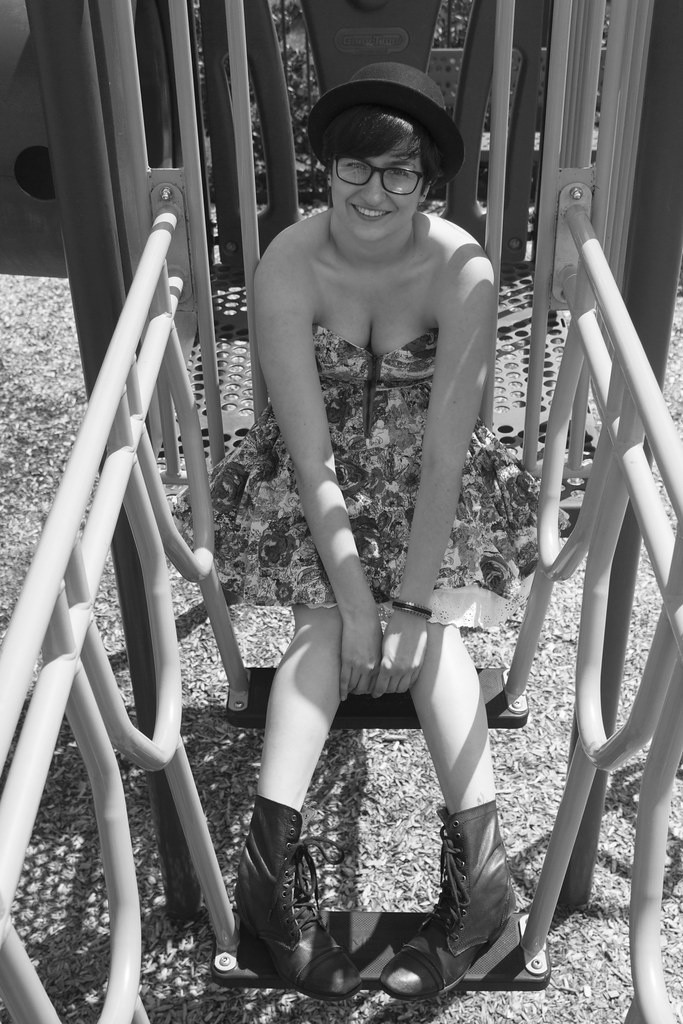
[
  {"xmin": 378, "ymin": 798, "xmax": 516, "ymax": 999},
  {"xmin": 233, "ymin": 794, "xmax": 363, "ymax": 1000}
]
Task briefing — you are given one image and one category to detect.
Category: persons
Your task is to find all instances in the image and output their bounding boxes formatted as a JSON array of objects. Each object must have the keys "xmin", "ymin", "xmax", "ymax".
[{"xmin": 170, "ymin": 61, "xmax": 569, "ymax": 1001}]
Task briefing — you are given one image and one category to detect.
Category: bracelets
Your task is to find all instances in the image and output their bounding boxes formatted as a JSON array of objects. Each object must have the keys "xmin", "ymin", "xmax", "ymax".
[{"xmin": 392, "ymin": 600, "xmax": 432, "ymax": 619}]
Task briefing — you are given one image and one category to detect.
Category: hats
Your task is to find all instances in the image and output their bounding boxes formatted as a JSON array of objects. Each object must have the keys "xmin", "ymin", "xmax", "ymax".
[{"xmin": 307, "ymin": 61, "xmax": 465, "ymax": 189}]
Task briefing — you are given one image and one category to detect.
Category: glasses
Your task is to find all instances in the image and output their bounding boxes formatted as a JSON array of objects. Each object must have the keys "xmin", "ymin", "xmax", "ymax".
[{"xmin": 332, "ymin": 153, "xmax": 425, "ymax": 195}]
